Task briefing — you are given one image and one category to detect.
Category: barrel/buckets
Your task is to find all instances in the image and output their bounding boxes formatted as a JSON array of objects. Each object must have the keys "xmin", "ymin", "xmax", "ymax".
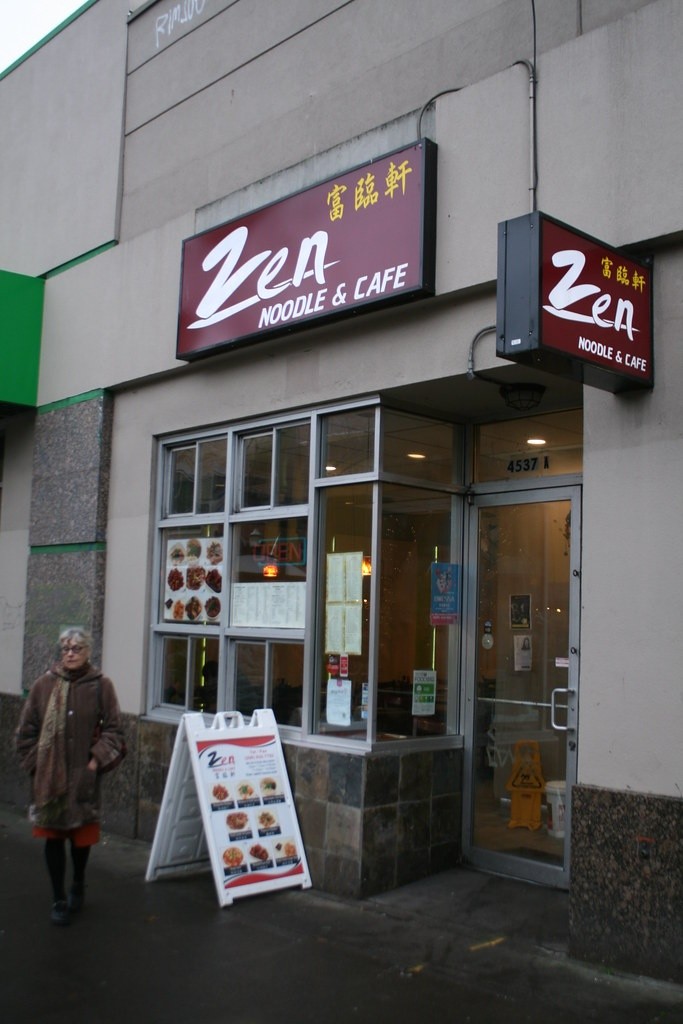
[{"xmin": 545, "ymin": 781, "xmax": 566, "ymax": 838}]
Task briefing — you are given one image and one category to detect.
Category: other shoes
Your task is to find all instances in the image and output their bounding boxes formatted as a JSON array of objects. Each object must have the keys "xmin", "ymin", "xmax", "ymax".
[
  {"xmin": 52, "ymin": 900, "xmax": 71, "ymax": 923},
  {"xmin": 70, "ymin": 886, "xmax": 86, "ymax": 909}
]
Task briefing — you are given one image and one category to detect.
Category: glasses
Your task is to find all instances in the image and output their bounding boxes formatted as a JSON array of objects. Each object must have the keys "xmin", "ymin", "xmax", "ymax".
[{"xmin": 59, "ymin": 645, "xmax": 88, "ymax": 654}]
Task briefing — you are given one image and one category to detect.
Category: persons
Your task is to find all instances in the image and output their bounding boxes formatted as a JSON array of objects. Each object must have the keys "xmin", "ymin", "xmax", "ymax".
[
  {"xmin": 520, "ymin": 638, "xmax": 531, "ymax": 651},
  {"xmin": 11, "ymin": 624, "xmax": 128, "ymax": 928},
  {"xmin": 163, "ymin": 652, "xmax": 296, "ymax": 723}
]
